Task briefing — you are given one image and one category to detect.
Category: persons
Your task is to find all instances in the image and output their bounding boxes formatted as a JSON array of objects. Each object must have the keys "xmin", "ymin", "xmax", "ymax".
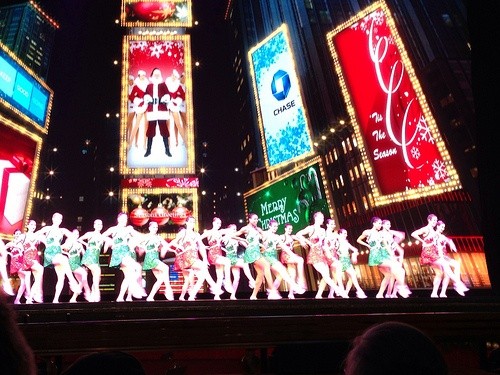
[
  {"xmin": 174, "ymin": 71, "xmax": 187, "ymax": 148},
  {"xmin": 1, "ymin": 212, "xmax": 470, "ymax": 304},
  {"xmin": 127, "ymin": 70, "xmax": 151, "ymax": 151},
  {"xmin": 143, "ymin": 67, "xmax": 174, "ymax": 157},
  {"xmin": 126, "ymin": 75, "xmax": 141, "ymax": 147},
  {"xmin": 164, "ymin": 67, "xmax": 187, "ymax": 148}
]
